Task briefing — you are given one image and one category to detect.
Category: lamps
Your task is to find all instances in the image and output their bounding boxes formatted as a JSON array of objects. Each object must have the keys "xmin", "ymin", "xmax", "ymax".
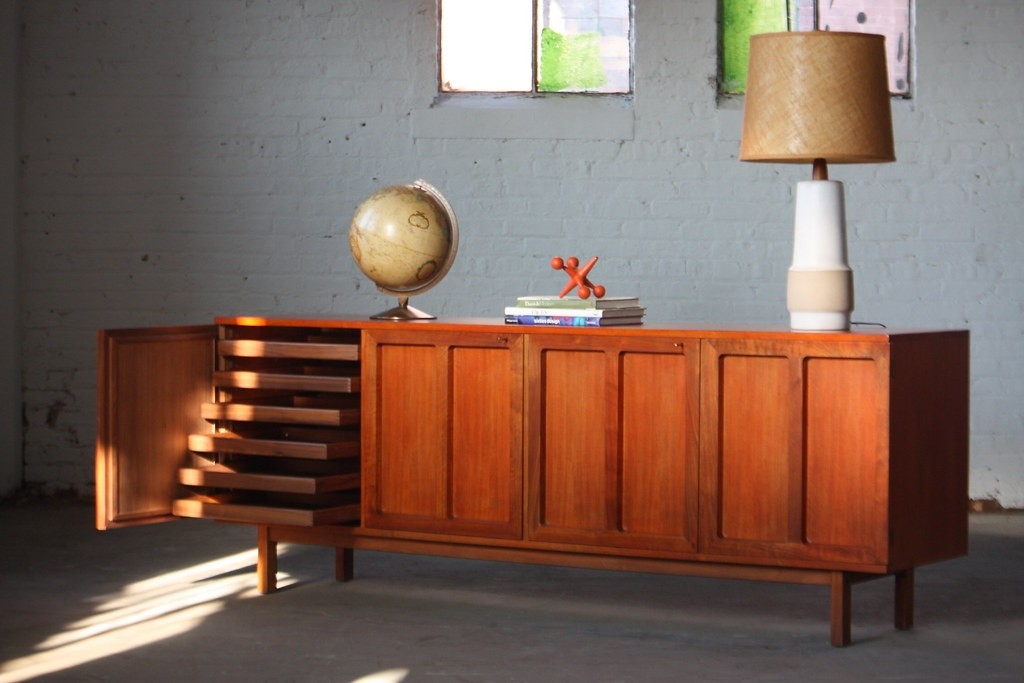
[{"xmin": 737, "ymin": 29, "xmax": 896, "ymax": 334}]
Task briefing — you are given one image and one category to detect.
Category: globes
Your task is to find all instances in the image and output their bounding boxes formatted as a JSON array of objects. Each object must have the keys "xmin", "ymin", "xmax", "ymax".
[{"xmin": 345, "ymin": 178, "xmax": 464, "ymax": 321}]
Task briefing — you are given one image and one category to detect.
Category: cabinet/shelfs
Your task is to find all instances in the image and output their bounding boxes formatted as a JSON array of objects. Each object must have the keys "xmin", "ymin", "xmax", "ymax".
[{"xmin": 91, "ymin": 316, "xmax": 971, "ymax": 648}]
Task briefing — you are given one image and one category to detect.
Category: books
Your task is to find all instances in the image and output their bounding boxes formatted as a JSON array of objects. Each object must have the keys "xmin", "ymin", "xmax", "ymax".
[{"xmin": 504, "ymin": 296, "xmax": 647, "ymax": 327}]
